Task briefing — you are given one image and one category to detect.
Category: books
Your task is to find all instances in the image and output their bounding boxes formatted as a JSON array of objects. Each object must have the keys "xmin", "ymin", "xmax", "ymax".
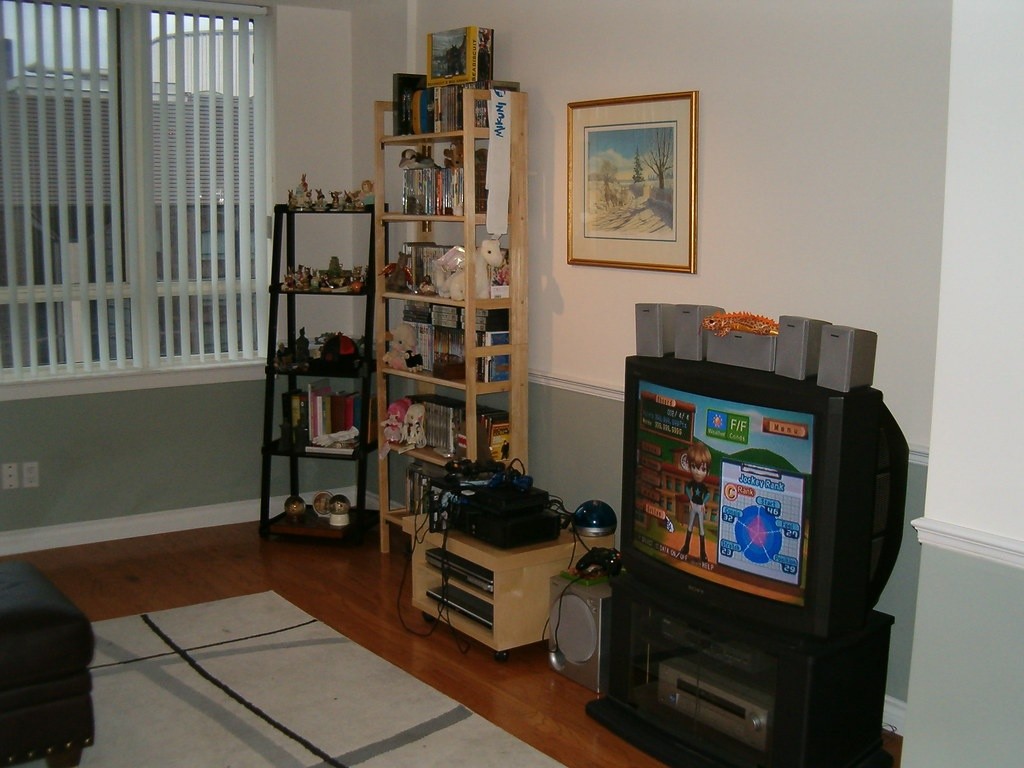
[
  {"xmin": 402, "ymin": 303, "xmax": 510, "ymax": 383},
  {"xmin": 402, "ymin": 241, "xmax": 510, "ymax": 300},
  {"xmin": 406, "ymin": 392, "xmax": 510, "ymax": 461},
  {"xmin": 403, "ymin": 167, "xmax": 488, "ymax": 217},
  {"xmin": 281, "ymin": 378, "xmax": 377, "ymax": 456},
  {"xmin": 435, "ymin": 80, "xmax": 488, "ymax": 133}
]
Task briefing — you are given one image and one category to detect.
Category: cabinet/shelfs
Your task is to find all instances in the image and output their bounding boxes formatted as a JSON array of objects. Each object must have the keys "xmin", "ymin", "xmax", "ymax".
[
  {"xmin": 585, "ymin": 578, "xmax": 895, "ymax": 768},
  {"xmin": 260, "ymin": 204, "xmax": 389, "ymax": 548},
  {"xmin": 401, "ymin": 514, "xmax": 614, "ymax": 662},
  {"xmin": 375, "ymin": 89, "xmax": 529, "ymax": 553}
]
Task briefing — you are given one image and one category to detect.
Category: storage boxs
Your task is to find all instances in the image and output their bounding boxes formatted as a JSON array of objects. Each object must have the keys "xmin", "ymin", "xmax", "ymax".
[
  {"xmin": 427, "ymin": 26, "xmax": 494, "ymax": 87},
  {"xmin": 434, "ymin": 361, "xmax": 465, "ymax": 380}
]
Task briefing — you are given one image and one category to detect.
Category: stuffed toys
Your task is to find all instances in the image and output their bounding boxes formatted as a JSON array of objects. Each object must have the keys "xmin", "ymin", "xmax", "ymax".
[
  {"xmin": 382, "ymin": 322, "xmax": 418, "ymax": 370},
  {"xmin": 385, "ymin": 251, "xmax": 412, "ymax": 294},
  {"xmin": 443, "ymin": 139, "xmax": 463, "ymax": 169},
  {"xmin": 399, "ymin": 150, "xmax": 438, "ymax": 169},
  {"xmin": 404, "ymin": 349, "xmax": 423, "ymax": 373},
  {"xmin": 381, "ymin": 398, "xmax": 427, "ymax": 449},
  {"xmin": 435, "ymin": 238, "xmax": 504, "ymax": 301}
]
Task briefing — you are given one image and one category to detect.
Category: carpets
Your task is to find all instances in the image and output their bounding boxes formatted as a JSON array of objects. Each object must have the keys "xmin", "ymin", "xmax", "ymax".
[{"xmin": 0, "ymin": 591, "xmax": 569, "ymax": 768}]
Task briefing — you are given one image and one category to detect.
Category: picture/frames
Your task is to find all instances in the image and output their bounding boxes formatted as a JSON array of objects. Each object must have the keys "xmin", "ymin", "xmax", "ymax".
[{"xmin": 567, "ymin": 90, "xmax": 699, "ymax": 276}]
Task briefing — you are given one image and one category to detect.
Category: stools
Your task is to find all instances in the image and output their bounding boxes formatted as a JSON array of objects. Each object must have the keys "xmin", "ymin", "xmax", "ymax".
[{"xmin": 0, "ymin": 559, "xmax": 94, "ymax": 768}]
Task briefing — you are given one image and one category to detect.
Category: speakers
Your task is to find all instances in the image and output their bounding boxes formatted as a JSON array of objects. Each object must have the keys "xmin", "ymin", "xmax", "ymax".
[
  {"xmin": 635, "ymin": 303, "xmax": 878, "ymax": 394},
  {"xmin": 546, "ymin": 575, "xmax": 615, "ymax": 694}
]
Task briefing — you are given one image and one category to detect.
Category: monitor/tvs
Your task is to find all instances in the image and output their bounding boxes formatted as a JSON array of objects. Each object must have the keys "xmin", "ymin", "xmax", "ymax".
[{"xmin": 620, "ymin": 354, "xmax": 910, "ymax": 641}]
